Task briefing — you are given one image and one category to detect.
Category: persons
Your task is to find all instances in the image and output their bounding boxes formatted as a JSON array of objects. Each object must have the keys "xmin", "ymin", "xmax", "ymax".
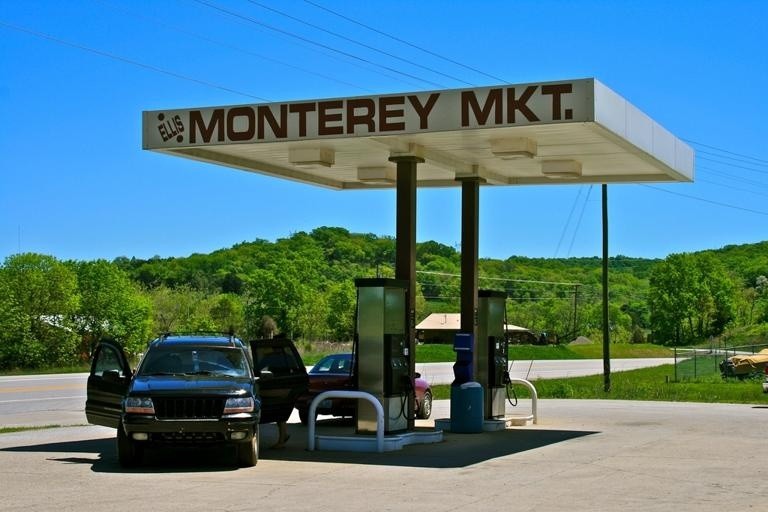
[{"xmin": 258, "ymin": 333, "xmax": 291, "ymax": 449}]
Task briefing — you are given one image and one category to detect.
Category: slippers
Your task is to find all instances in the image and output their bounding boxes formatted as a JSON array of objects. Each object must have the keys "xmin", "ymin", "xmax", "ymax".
[{"xmin": 269, "ymin": 441, "xmax": 294, "ymax": 451}]
